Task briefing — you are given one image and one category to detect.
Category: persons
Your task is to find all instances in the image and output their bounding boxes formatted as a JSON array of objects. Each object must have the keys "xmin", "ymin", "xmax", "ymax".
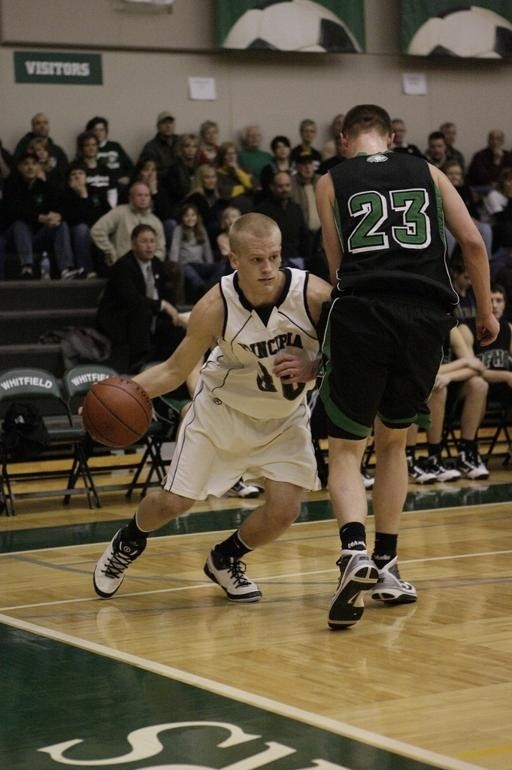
[
  {"xmin": 390, "ymin": 116, "xmax": 499, "ymax": 257},
  {"xmin": 446, "ymin": 260, "xmax": 482, "ymax": 337},
  {"xmin": 303, "ymin": 109, "xmax": 347, "ymax": 211},
  {"xmin": 0, "ymin": 112, "xmax": 78, "ymax": 281},
  {"xmin": 178, "ymin": 348, "xmax": 259, "ymax": 498},
  {"xmin": 304, "ymin": 103, "xmax": 500, "ymax": 629},
  {"xmin": 360, "ymin": 394, "xmax": 437, "ymax": 491},
  {"xmin": 427, "ymin": 311, "xmax": 491, "ymax": 482},
  {"xmin": 78, "ymin": 111, "xmax": 302, "ymax": 211},
  {"xmin": 77, "ymin": 212, "xmax": 332, "ymax": 604},
  {"xmin": 500, "ymin": 130, "xmax": 511, "ymax": 257},
  {"xmin": 464, "ymin": 284, "xmax": 512, "ymax": 469},
  {"xmin": 78, "ymin": 213, "xmax": 259, "ymax": 342}
]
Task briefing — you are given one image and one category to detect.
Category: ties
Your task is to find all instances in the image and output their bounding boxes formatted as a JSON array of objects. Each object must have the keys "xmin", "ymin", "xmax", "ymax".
[{"xmin": 146, "ymin": 265, "xmax": 156, "ymax": 334}]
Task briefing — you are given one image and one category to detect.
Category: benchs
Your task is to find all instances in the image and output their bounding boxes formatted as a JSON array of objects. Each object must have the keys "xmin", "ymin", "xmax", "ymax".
[{"xmin": 0, "ymin": 276, "xmax": 109, "ymax": 385}]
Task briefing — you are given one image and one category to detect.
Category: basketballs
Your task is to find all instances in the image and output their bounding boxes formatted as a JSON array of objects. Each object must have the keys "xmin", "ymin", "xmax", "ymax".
[{"xmin": 84, "ymin": 379, "xmax": 152, "ymax": 448}]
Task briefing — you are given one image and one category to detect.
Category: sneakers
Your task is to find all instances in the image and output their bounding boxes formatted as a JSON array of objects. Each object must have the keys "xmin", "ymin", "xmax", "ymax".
[
  {"xmin": 227, "ymin": 482, "xmax": 259, "ymax": 498},
  {"xmin": 457, "ymin": 451, "xmax": 489, "ymax": 479},
  {"xmin": 408, "ymin": 456, "xmax": 436, "ymax": 485},
  {"xmin": 327, "ymin": 549, "xmax": 379, "ymax": 630},
  {"xmin": 426, "ymin": 456, "xmax": 461, "ymax": 482},
  {"xmin": 93, "ymin": 527, "xmax": 146, "ymax": 598},
  {"xmin": 204, "ymin": 547, "xmax": 262, "ymax": 602},
  {"xmin": 372, "ymin": 555, "xmax": 416, "ymax": 603},
  {"xmin": 360, "ymin": 470, "xmax": 375, "ymax": 488}
]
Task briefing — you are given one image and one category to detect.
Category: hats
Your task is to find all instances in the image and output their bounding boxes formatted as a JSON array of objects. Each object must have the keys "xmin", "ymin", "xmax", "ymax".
[{"xmin": 157, "ymin": 111, "xmax": 174, "ymax": 124}]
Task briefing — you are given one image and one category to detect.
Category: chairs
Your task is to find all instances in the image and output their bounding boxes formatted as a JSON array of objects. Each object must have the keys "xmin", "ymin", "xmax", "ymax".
[
  {"xmin": 314, "ymin": 391, "xmax": 511, "ymax": 483},
  {"xmin": 0, "ymin": 368, "xmax": 95, "ymax": 516},
  {"xmin": 64, "ymin": 365, "xmax": 166, "ymax": 509}
]
